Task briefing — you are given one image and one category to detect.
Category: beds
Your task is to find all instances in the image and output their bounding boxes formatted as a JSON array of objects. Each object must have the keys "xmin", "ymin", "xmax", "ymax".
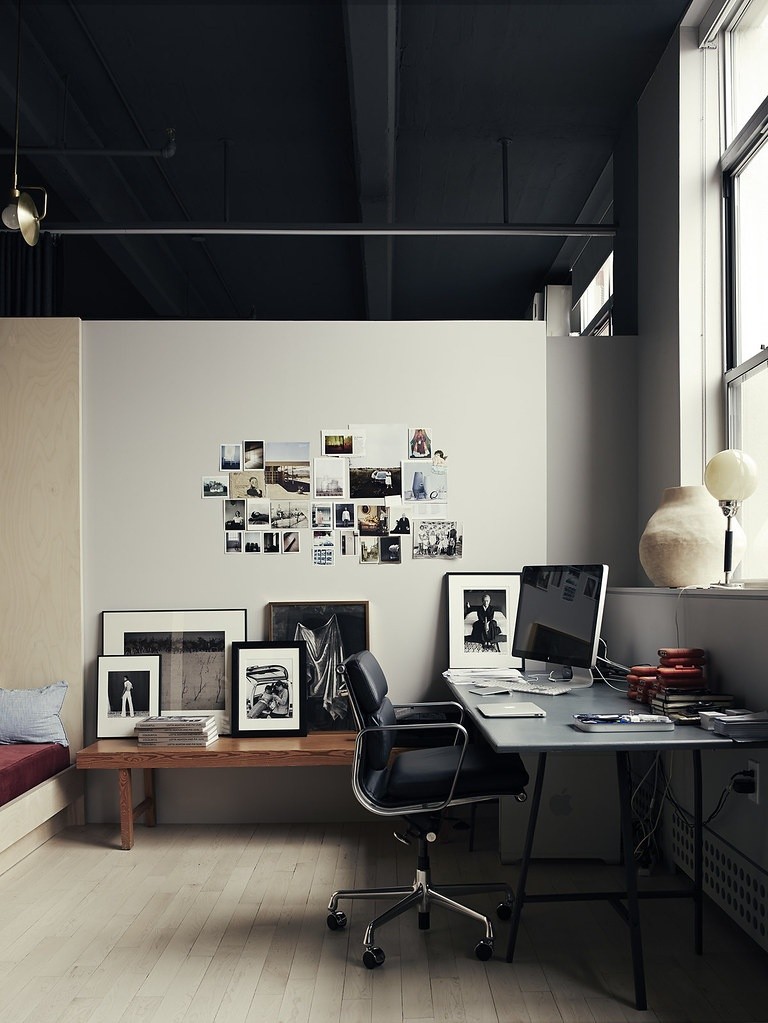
[{"xmin": 0, "ymin": 744, "xmax": 85, "ymax": 854}]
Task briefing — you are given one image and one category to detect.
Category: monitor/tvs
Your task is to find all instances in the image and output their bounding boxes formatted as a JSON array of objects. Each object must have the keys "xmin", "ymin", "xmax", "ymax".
[{"xmin": 512, "ymin": 563, "xmax": 609, "ymax": 688}]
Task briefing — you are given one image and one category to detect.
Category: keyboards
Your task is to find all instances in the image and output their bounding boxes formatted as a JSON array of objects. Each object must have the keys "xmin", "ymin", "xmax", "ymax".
[{"xmin": 473, "ymin": 679, "xmax": 572, "ymax": 696}]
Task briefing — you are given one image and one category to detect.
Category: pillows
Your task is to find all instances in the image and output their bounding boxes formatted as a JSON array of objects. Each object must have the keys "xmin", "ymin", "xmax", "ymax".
[{"xmin": 0, "ymin": 680, "xmax": 70, "ymax": 748}]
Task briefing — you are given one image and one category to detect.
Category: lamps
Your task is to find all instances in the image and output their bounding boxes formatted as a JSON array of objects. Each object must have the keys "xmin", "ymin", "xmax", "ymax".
[
  {"xmin": 703, "ymin": 449, "xmax": 759, "ymax": 588},
  {"xmin": 2, "ymin": 0, "xmax": 49, "ymax": 246}
]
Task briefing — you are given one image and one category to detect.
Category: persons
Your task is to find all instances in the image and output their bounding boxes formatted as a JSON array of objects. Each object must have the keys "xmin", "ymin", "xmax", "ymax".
[
  {"xmin": 226, "ymin": 511, "xmax": 243, "ymax": 525},
  {"xmin": 247, "ymin": 477, "xmax": 262, "ymax": 498},
  {"xmin": 477, "ymin": 595, "xmax": 497, "ymax": 649},
  {"xmin": 379, "ymin": 510, "xmax": 388, "ymax": 532},
  {"xmin": 121, "ymin": 675, "xmax": 134, "ymax": 718},
  {"xmin": 399, "ymin": 512, "xmax": 410, "ymax": 528},
  {"xmin": 342, "ymin": 507, "xmax": 350, "ymax": 527},
  {"xmin": 318, "ymin": 511, "xmax": 323, "ymax": 525},
  {"xmin": 413, "ymin": 429, "xmax": 430, "ymax": 457},
  {"xmin": 371, "ymin": 470, "xmax": 378, "ymax": 483},
  {"xmin": 246, "ymin": 679, "xmax": 288, "ymax": 719},
  {"xmin": 276, "ymin": 504, "xmax": 284, "ymax": 520},
  {"xmin": 385, "ymin": 469, "xmax": 393, "ymax": 489},
  {"xmin": 418, "ymin": 524, "xmax": 458, "ymax": 557}
]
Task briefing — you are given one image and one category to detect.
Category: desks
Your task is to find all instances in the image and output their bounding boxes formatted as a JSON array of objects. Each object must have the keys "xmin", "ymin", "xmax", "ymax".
[
  {"xmin": 441, "ymin": 670, "xmax": 768, "ymax": 1011},
  {"xmin": 76, "ymin": 731, "xmax": 447, "ymax": 850}
]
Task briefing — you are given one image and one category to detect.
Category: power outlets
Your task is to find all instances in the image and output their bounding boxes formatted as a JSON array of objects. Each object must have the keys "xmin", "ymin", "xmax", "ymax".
[{"xmin": 746, "ymin": 759, "xmax": 759, "ymax": 803}]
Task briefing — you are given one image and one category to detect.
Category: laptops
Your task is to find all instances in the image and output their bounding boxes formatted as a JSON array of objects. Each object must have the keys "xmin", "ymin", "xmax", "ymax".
[{"xmin": 477, "ymin": 702, "xmax": 546, "ymax": 717}]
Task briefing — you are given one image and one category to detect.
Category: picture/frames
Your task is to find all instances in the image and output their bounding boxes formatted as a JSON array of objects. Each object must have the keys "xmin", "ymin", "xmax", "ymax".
[
  {"xmin": 232, "ymin": 641, "xmax": 308, "ymax": 738},
  {"xmin": 96, "ymin": 654, "xmax": 162, "ymax": 740},
  {"xmin": 445, "ymin": 571, "xmax": 525, "ymax": 672},
  {"xmin": 268, "ymin": 601, "xmax": 369, "ymax": 734},
  {"xmin": 101, "ymin": 609, "xmax": 248, "ymax": 738}
]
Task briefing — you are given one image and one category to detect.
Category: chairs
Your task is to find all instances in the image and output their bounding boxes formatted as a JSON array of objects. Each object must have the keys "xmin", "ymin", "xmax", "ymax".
[{"xmin": 327, "ymin": 650, "xmax": 529, "ymax": 970}]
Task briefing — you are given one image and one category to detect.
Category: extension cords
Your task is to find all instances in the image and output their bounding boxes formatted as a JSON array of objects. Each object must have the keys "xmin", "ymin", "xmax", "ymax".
[{"xmin": 638, "ymin": 862, "xmax": 655, "ymax": 875}]
